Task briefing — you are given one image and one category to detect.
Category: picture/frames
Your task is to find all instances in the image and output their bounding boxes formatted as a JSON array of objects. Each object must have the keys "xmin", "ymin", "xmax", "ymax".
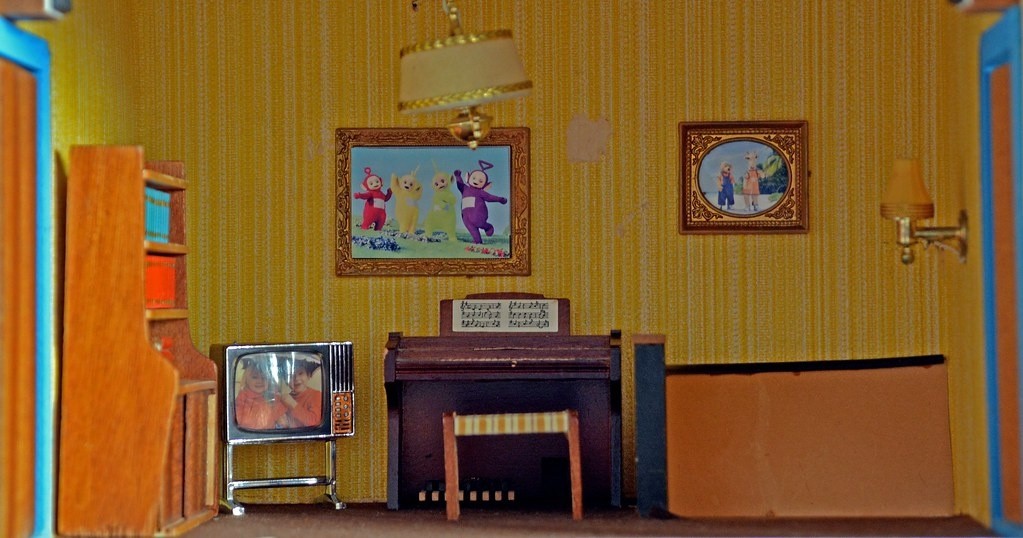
[
  {"xmin": 334, "ymin": 126, "xmax": 531, "ymax": 277},
  {"xmin": 678, "ymin": 120, "xmax": 810, "ymax": 235}
]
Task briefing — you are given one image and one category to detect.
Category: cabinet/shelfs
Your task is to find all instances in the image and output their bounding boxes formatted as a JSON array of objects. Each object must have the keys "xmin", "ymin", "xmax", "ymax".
[{"xmin": 54, "ymin": 146, "xmax": 220, "ymax": 538}]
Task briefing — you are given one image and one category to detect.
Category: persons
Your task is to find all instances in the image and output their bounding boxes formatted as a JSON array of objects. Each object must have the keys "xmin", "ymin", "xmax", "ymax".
[{"xmin": 235, "ymin": 351, "xmax": 322, "ymax": 430}]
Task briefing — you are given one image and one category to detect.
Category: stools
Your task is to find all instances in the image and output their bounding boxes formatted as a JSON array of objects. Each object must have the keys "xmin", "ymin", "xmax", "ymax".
[{"xmin": 441, "ymin": 410, "xmax": 583, "ymax": 520}]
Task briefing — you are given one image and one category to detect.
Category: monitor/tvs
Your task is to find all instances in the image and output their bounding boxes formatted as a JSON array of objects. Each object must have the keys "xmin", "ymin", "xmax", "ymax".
[{"xmin": 220, "ymin": 339, "xmax": 355, "ymax": 443}]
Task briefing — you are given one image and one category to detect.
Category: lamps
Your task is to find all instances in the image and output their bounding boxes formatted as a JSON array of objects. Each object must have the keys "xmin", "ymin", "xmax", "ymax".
[
  {"xmin": 398, "ymin": 0, "xmax": 533, "ymax": 149},
  {"xmin": 880, "ymin": 158, "xmax": 968, "ymax": 264}
]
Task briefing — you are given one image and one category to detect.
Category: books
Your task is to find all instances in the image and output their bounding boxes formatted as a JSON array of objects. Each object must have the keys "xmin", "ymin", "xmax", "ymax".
[
  {"xmin": 144, "ymin": 186, "xmax": 171, "ymax": 242},
  {"xmin": 144, "ymin": 255, "xmax": 178, "ymax": 309}
]
